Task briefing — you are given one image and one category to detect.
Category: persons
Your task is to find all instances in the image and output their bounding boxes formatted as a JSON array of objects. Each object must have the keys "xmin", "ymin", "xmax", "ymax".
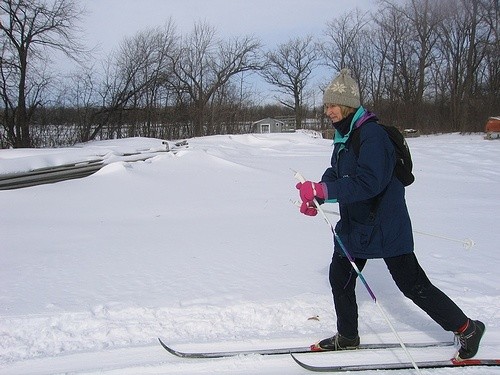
[{"xmin": 296, "ymin": 69, "xmax": 485, "ymax": 361}]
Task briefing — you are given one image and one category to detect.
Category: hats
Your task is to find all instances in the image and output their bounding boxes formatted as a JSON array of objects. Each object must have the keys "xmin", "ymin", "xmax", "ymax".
[{"xmin": 323, "ymin": 68, "xmax": 360, "ymax": 108}]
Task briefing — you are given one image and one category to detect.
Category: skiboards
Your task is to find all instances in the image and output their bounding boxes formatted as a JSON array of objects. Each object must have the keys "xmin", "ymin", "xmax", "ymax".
[
  {"xmin": 159, "ymin": 337, "xmax": 455, "ymax": 358},
  {"xmin": 288, "ymin": 351, "xmax": 500, "ymax": 372}
]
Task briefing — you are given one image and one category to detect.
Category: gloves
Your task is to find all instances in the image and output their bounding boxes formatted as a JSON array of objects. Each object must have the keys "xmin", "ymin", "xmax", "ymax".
[
  {"xmin": 296, "ymin": 180, "xmax": 324, "ymax": 202},
  {"xmin": 300, "ymin": 202, "xmax": 317, "ymax": 216}
]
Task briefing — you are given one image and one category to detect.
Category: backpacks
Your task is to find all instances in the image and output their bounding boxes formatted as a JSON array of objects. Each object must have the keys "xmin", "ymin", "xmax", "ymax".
[{"xmin": 352, "ymin": 120, "xmax": 415, "ymax": 187}]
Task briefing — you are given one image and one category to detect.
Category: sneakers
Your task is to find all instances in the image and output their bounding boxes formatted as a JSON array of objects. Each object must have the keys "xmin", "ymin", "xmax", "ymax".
[
  {"xmin": 455, "ymin": 318, "xmax": 485, "ymax": 359},
  {"xmin": 316, "ymin": 332, "xmax": 360, "ymax": 349}
]
